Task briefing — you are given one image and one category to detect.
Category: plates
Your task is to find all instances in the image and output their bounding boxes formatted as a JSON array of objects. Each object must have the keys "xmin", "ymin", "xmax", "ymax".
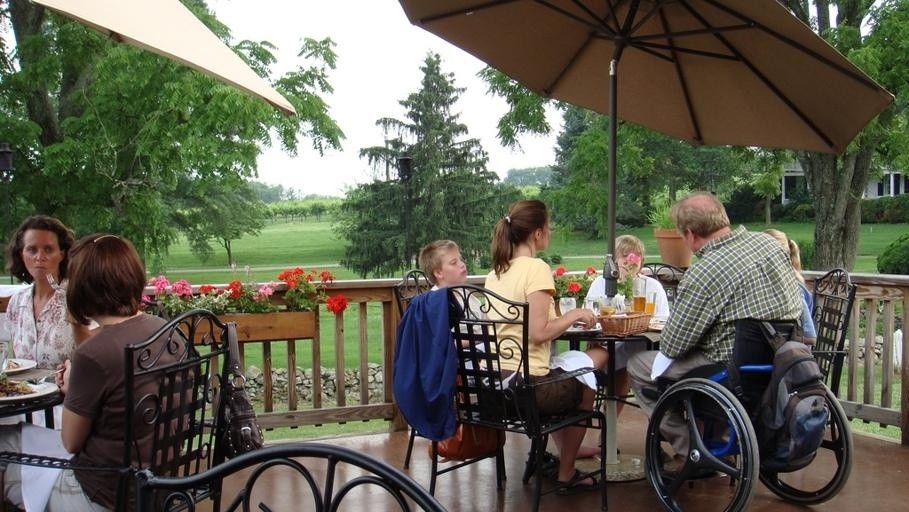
[{"xmin": 1, "ymin": 358, "xmax": 38, "ymax": 375}]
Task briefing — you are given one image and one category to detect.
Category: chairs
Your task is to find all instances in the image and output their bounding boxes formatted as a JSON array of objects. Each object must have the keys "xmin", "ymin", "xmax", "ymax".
[
  {"xmin": 393, "ymin": 269, "xmax": 507, "ymax": 479},
  {"xmin": 0, "ymin": 308, "xmax": 238, "ymax": 512},
  {"xmin": 815, "ymin": 267, "xmax": 857, "ymax": 391},
  {"xmin": 134, "ymin": 441, "xmax": 449, "ymax": 512},
  {"xmin": 641, "ymin": 262, "xmax": 686, "ymax": 313},
  {"xmin": 429, "ymin": 284, "xmax": 608, "ymax": 512}
]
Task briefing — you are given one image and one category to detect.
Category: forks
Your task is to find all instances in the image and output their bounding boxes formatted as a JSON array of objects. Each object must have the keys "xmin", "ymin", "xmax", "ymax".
[
  {"xmin": 46, "ymin": 273, "xmax": 67, "ymax": 300},
  {"xmin": 53, "ymin": 368, "xmax": 66, "ymax": 374}
]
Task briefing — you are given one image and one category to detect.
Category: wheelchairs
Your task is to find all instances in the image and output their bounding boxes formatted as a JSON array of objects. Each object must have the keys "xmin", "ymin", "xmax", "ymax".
[{"xmin": 642, "ymin": 321, "xmax": 852, "ymax": 511}]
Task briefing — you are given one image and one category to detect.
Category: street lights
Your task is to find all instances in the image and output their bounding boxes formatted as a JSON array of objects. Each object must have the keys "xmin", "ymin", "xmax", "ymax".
[{"xmin": 396, "ymin": 149, "xmax": 413, "ymax": 271}]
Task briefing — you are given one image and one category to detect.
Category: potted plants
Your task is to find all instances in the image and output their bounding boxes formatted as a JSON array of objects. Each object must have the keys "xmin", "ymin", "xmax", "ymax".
[{"xmin": 646, "ymin": 183, "xmax": 697, "ymax": 268}]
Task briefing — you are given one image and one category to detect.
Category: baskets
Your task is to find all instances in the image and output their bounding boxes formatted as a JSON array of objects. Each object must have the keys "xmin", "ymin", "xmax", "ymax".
[{"xmin": 596, "ymin": 312, "xmax": 653, "ymax": 334}]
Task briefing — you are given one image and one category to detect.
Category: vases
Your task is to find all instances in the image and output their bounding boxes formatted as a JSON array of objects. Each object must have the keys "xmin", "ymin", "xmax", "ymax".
[{"xmin": 559, "ymin": 297, "xmax": 576, "ymax": 315}]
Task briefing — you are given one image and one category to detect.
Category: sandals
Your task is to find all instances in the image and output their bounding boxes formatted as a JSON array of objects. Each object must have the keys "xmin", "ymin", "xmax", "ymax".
[
  {"xmin": 557, "ymin": 469, "xmax": 604, "ymax": 495},
  {"xmin": 526, "ymin": 452, "xmax": 561, "ymax": 479}
]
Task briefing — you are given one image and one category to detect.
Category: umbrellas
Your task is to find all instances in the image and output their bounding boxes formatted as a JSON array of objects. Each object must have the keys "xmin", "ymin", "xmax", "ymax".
[
  {"xmin": 0, "ymin": 3, "xmax": 296, "ymax": 116},
  {"xmin": 398, "ymin": 0, "xmax": 894, "ymax": 296}
]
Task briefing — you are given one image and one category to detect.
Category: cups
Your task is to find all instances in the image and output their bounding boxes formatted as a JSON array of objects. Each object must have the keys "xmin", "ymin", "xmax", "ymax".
[
  {"xmin": 559, "ymin": 297, "xmax": 576, "ymax": 332},
  {"xmin": 600, "ymin": 297, "xmax": 617, "ymax": 315},
  {"xmin": 633, "ymin": 279, "xmax": 646, "ymax": 314},
  {"xmin": 646, "ymin": 292, "xmax": 656, "ymax": 315},
  {"xmin": 0, "ymin": 342, "xmax": 8, "ymax": 375}
]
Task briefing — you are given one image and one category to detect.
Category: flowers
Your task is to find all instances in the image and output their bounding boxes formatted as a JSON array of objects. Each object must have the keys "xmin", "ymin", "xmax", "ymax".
[
  {"xmin": 139, "ymin": 266, "xmax": 349, "ymax": 317},
  {"xmin": 615, "ymin": 253, "xmax": 643, "ymax": 301},
  {"xmin": 551, "ymin": 267, "xmax": 597, "ymax": 301}
]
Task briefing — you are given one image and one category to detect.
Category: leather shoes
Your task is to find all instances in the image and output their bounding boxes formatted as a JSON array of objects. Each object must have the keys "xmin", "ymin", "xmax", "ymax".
[{"xmin": 664, "ymin": 456, "xmax": 687, "ymax": 471}]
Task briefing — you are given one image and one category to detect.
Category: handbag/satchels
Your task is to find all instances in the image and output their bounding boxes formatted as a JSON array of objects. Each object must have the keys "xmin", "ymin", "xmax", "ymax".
[
  {"xmin": 428, "ymin": 425, "xmax": 505, "ymax": 459},
  {"xmin": 224, "ymin": 321, "xmax": 264, "ymax": 459}
]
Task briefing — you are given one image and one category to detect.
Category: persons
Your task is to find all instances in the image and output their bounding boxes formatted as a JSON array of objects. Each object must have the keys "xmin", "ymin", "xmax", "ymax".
[
  {"xmin": 5, "ymin": 235, "xmax": 195, "ymax": 511},
  {"xmin": 765, "ymin": 226, "xmax": 823, "ymax": 352},
  {"xmin": 7, "ymin": 217, "xmax": 76, "ymax": 374},
  {"xmin": 419, "ymin": 239, "xmax": 484, "ymax": 352},
  {"xmin": 482, "ymin": 201, "xmax": 610, "ymax": 489},
  {"xmin": 583, "ymin": 234, "xmax": 669, "ymax": 440},
  {"xmin": 628, "ymin": 193, "xmax": 804, "ymax": 477}
]
